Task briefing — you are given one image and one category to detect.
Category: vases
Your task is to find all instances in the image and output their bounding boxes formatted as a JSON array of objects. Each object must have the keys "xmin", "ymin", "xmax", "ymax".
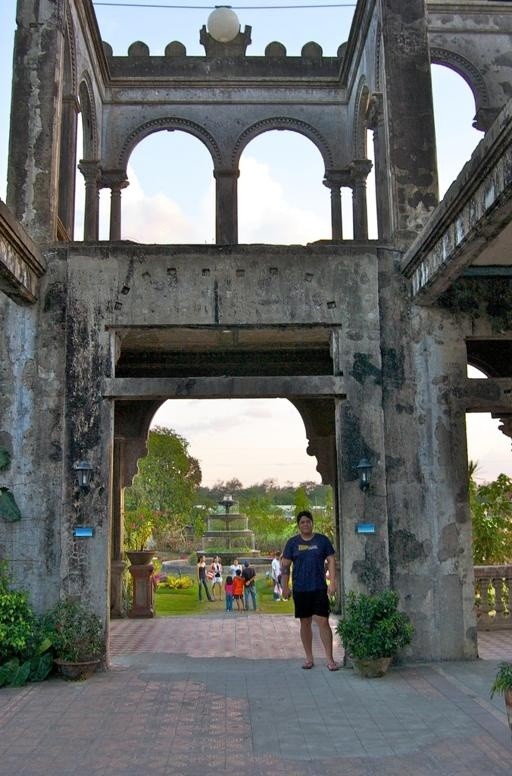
[{"xmin": 122, "ymin": 503, "xmax": 157, "ymax": 565}]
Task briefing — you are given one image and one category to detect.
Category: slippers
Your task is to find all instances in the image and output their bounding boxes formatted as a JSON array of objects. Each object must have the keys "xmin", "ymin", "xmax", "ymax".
[
  {"xmin": 327, "ymin": 663, "xmax": 339, "ymax": 671},
  {"xmin": 303, "ymin": 662, "xmax": 314, "ymax": 669}
]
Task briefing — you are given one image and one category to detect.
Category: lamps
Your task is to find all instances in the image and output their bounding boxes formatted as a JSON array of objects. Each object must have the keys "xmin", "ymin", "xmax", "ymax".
[
  {"xmin": 356, "ymin": 458, "xmax": 376, "ymax": 492},
  {"xmin": 75, "ymin": 460, "xmax": 94, "ymax": 497},
  {"xmin": 207, "ymin": 6, "xmax": 240, "ymax": 44}
]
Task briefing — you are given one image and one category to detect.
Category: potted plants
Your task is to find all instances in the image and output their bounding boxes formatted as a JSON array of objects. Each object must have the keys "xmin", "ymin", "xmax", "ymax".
[
  {"xmin": 40, "ymin": 598, "xmax": 104, "ymax": 683},
  {"xmin": 488, "ymin": 661, "xmax": 512, "ymax": 731},
  {"xmin": 333, "ymin": 589, "xmax": 416, "ymax": 679}
]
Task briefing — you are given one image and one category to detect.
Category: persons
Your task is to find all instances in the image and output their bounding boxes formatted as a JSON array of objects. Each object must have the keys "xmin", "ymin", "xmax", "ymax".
[
  {"xmin": 196, "ymin": 555, "xmax": 257, "ymax": 612},
  {"xmin": 281, "ymin": 510, "xmax": 338, "ymax": 671},
  {"xmin": 272, "ymin": 552, "xmax": 282, "ymax": 601}
]
